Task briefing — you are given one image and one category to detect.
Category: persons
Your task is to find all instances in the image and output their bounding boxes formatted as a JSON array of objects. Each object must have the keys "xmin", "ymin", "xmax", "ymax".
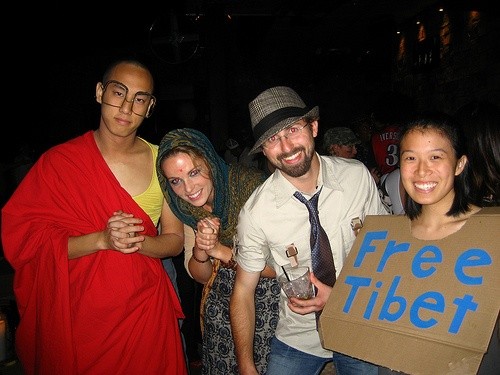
[
  {"xmin": 323, "ymin": 126, "xmax": 380, "ymax": 184},
  {"xmin": 230, "ymin": 86, "xmax": 390, "ymax": 375},
  {"xmin": 1, "ymin": 59, "xmax": 189, "ymax": 375},
  {"xmin": 377, "ymin": 113, "xmax": 500, "ymax": 375},
  {"xmin": 357, "ymin": 112, "xmax": 405, "ymax": 216},
  {"xmin": 155, "ymin": 128, "xmax": 281, "ymax": 375}
]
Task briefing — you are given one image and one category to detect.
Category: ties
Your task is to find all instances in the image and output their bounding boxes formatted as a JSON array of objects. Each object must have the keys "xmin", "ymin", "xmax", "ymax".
[{"xmin": 293, "ymin": 186, "xmax": 336, "ymax": 331}]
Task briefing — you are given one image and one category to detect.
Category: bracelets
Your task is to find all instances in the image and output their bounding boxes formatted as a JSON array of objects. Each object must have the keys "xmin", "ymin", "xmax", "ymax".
[
  {"xmin": 221, "ymin": 252, "xmax": 238, "ymax": 271},
  {"xmin": 192, "ymin": 247, "xmax": 210, "ymax": 263}
]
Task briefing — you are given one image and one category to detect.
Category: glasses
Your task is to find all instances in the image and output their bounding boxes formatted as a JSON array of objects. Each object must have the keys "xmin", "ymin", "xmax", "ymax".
[{"xmin": 101, "ymin": 80, "xmax": 156, "ymax": 118}]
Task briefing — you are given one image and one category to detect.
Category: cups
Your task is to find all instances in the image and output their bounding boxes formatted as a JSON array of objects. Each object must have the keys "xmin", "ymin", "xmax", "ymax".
[{"xmin": 277, "ymin": 266, "xmax": 313, "ymax": 306}]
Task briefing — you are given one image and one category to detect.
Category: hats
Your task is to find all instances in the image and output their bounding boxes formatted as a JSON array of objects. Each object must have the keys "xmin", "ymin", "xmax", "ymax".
[
  {"xmin": 322, "ymin": 127, "xmax": 361, "ymax": 149},
  {"xmin": 247, "ymin": 87, "xmax": 319, "ymax": 156}
]
